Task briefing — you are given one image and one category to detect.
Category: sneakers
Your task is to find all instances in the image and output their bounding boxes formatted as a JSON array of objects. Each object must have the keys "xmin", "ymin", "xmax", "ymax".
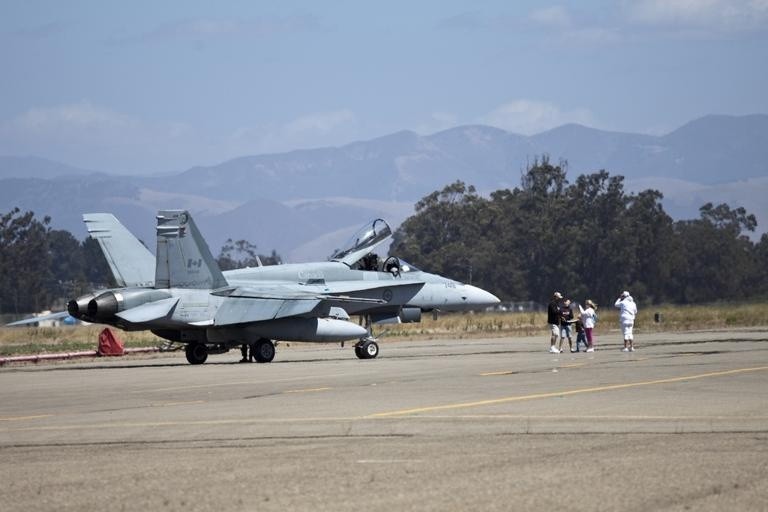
[
  {"xmin": 583, "ymin": 348, "xmax": 595, "ymax": 353},
  {"xmin": 622, "ymin": 347, "xmax": 635, "ymax": 352},
  {"xmin": 570, "ymin": 348, "xmax": 580, "ymax": 353},
  {"xmin": 549, "ymin": 347, "xmax": 565, "ymax": 353}
]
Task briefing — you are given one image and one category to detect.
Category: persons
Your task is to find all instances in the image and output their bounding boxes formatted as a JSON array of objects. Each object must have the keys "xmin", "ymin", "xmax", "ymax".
[
  {"xmin": 548, "ymin": 291, "xmax": 600, "ymax": 354},
  {"xmin": 614, "ymin": 290, "xmax": 639, "ymax": 352}
]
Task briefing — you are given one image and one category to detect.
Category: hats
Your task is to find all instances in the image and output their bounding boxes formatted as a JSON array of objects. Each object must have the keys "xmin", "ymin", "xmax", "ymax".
[
  {"xmin": 553, "ymin": 292, "xmax": 563, "ymax": 299},
  {"xmin": 620, "ymin": 291, "xmax": 630, "ymax": 296}
]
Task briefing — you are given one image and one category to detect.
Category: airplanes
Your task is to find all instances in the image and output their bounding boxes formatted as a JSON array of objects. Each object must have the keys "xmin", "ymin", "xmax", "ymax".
[
  {"xmin": 6, "ymin": 311, "xmax": 68, "ymax": 326},
  {"xmin": 68, "ymin": 209, "xmax": 502, "ymax": 365}
]
